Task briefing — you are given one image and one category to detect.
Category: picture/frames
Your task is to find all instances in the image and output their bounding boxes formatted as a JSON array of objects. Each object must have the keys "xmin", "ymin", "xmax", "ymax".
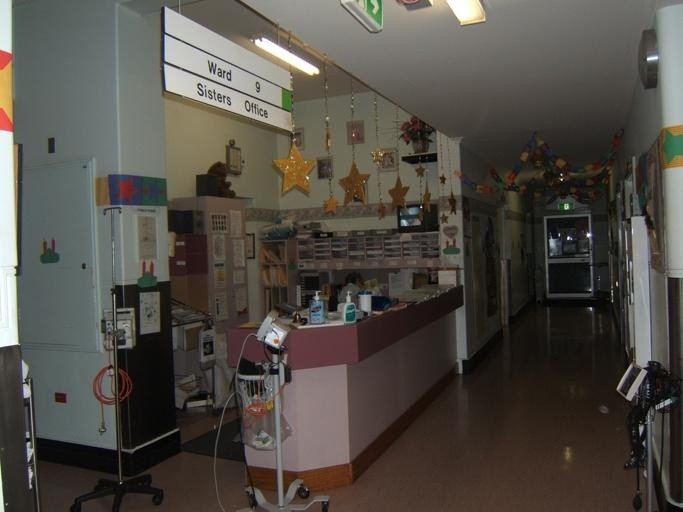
[{"xmin": 288, "ymin": 117, "xmax": 400, "ymax": 210}]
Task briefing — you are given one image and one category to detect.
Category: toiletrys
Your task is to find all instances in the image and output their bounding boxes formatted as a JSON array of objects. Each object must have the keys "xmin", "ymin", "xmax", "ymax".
[
  {"xmin": 308, "ymin": 290, "xmax": 327, "ymax": 325},
  {"xmin": 342, "ymin": 290, "xmax": 357, "ymax": 325}
]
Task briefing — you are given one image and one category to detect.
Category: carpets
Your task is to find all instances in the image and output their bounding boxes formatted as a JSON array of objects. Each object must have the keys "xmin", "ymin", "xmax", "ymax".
[{"xmin": 181, "ymin": 417, "xmax": 246, "ymax": 463}]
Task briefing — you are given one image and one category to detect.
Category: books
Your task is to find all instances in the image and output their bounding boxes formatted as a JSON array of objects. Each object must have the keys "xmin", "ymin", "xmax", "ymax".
[{"xmin": 172, "ymin": 303, "xmax": 216, "ymax": 387}]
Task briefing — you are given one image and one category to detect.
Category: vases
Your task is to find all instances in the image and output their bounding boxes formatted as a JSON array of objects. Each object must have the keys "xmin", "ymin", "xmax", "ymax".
[{"xmin": 412, "ymin": 135, "xmax": 429, "ymax": 153}]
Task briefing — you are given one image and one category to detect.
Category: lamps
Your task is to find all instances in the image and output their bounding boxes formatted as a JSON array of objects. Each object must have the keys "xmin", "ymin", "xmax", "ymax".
[
  {"xmin": 252, "ymin": 33, "xmax": 322, "ymax": 80},
  {"xmin": 446, "ymin": 0, "xmax": 486, "ymax": 27}
]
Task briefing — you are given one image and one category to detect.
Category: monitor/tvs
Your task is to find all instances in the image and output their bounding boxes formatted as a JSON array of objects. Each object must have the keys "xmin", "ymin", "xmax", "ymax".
[{"xmin": 412, "ymin": 272, "xmax": 431, "ymax": 290}]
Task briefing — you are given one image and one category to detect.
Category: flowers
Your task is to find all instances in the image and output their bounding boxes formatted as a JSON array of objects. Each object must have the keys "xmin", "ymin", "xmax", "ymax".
[{"xmin": 397, "ymin": 114, "xmax": 434, "ymax": 147}]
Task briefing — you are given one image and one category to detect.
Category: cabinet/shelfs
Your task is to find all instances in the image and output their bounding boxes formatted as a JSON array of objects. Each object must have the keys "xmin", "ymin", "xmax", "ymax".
[
  {"xmin": 295, "ymin": 231, "xmax": 441, "ymax": 270},
  {"xmin": 260, "ymin": 238, "xmax": 298, "ymax": 316}
]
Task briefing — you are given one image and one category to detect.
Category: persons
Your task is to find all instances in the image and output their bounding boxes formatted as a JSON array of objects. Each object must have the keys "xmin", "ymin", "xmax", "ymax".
[{"xmin": 339, "ymin": 272, "xmax": 380, "ymax": 313}]
